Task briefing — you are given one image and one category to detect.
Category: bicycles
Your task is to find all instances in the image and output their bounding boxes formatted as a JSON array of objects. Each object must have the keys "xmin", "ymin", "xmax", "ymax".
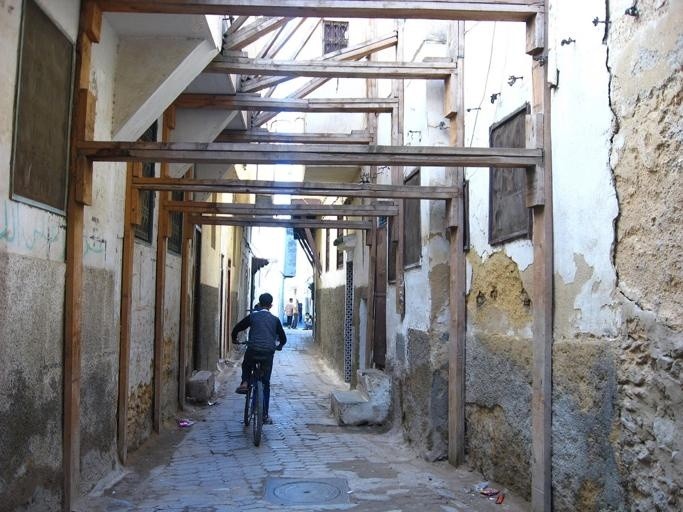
[{"xmin": 234, "ymin": 341, "xmax": 283, "ymax": 448}]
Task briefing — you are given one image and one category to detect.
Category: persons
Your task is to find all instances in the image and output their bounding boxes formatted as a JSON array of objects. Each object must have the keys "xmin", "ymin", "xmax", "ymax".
[
  {"xmin": 231, "ymin": 292, "xmax": 287, "ymax": 425},
  {"xmin": 285, "ymin": 297, "xmax": 316, "ymax": 330}
]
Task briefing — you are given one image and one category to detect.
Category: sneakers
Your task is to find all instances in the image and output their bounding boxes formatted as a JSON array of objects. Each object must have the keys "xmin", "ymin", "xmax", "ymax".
[
  {"xmin": 262, "ymin": 417, "xmax": 272, "ymax": 425},
  {"xmin": 235, "ymin": 387, "xmax": 245, "ymax": 393}
]
